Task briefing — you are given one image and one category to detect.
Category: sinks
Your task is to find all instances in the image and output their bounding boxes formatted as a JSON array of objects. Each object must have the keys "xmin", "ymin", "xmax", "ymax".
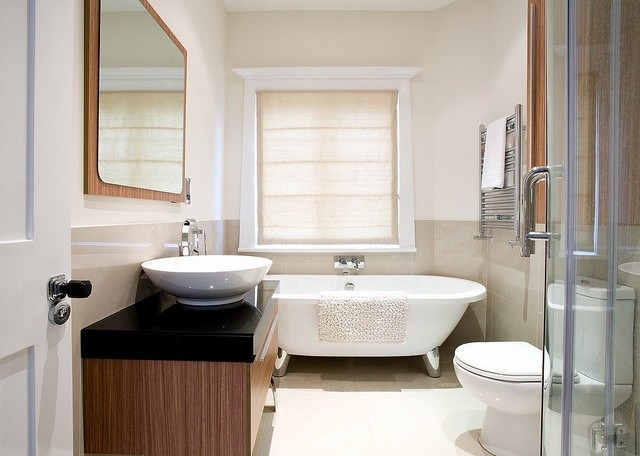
[
  {"xmin": 618, "ymin": 262, "xmax": 640, "ymax": 290},
  {"xmin": 141, "ymin": 254, "xmax": 272, "ymax": 306}
]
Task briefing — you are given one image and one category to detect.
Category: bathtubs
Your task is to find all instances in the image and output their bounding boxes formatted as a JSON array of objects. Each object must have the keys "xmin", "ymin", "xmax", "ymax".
[{"xmin": 262, "ymin": 274, "xmax": 486, "ymax": 378}]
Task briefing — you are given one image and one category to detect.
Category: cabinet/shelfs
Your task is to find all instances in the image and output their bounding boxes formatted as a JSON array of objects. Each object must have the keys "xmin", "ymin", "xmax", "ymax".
[
  {"xmin": 80, "ymin": 278, "xmax": 281, "ymax": 454},
  {"xmin": 526, "ymin": 2, "xmax": 550, "ymax": 224}
]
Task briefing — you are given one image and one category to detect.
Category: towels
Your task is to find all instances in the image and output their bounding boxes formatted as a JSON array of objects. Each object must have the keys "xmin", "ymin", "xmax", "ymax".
[
  {"xmin": 317, "ymin": 293, "xmax": 406, "ymax": 342},
  {"xmin": 480, "ymin": 116, "xmax": 507, "ymax": 194}
]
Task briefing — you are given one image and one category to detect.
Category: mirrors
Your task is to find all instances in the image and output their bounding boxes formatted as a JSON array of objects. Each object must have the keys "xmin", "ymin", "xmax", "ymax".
[{"xmin": 85, "ymin": 0, "xmax": 187, "ymax": 204}]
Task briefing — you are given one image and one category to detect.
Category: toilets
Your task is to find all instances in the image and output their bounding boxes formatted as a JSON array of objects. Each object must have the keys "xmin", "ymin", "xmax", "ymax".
[{"xmin": 453, "ymin": 276, "xmax": 637, "ymax": 454}]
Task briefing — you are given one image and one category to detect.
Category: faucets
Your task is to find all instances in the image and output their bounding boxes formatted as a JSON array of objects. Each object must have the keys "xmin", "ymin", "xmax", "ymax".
[
  {"xmin": 179, "ymin": 218, "xmax": 200, "ymax": 255},
  {"xmin": 351, "ymin": 257, "xmax": 360, "ymax": 272}
]
